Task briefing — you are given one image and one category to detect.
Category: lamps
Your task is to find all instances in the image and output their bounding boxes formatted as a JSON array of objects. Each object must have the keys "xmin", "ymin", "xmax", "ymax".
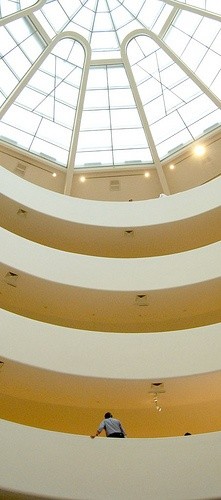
[{"xmin": 153, "ymin": 393, "xmax": 163, "ymax": 413}]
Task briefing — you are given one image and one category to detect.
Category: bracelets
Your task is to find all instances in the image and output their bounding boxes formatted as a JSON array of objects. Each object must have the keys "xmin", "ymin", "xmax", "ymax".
[{"xmin": 96, "ymin": 434, "xmax": 98, "ymax": 436}]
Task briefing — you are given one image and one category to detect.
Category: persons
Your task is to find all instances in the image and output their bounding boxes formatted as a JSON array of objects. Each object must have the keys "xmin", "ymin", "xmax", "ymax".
[{"xmin": 90, "ymin": 411, "xmax": 126, "ymax": 439}]
